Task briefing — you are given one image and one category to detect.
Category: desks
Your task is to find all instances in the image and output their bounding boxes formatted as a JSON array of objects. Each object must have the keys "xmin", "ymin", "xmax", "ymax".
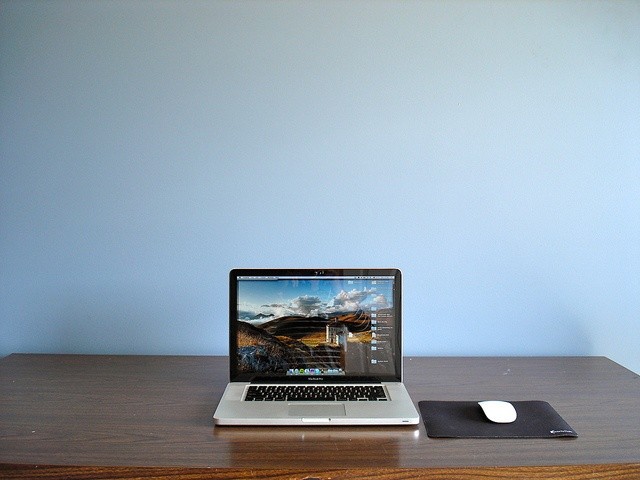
[{"xmin": 0, "ymin": 353, "xmax": 640, "ymax": 480}]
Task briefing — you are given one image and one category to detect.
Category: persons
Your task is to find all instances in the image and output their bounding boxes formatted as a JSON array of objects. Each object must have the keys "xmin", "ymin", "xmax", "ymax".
[{"xmin": 260, "ymin": 270, "xmax": 378, "ymax": 373}]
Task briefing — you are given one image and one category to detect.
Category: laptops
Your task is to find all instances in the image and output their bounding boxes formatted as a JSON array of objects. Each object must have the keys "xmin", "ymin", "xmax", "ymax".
[{"xmin": 212, "ymin": 268, "xmax": 420, "ymax": 427}]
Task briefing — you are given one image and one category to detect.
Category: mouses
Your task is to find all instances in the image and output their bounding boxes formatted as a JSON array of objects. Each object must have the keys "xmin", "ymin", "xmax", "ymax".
[{"xmin": 477, "ymin": 399, "xmax": 517, "ymax": 424}]
